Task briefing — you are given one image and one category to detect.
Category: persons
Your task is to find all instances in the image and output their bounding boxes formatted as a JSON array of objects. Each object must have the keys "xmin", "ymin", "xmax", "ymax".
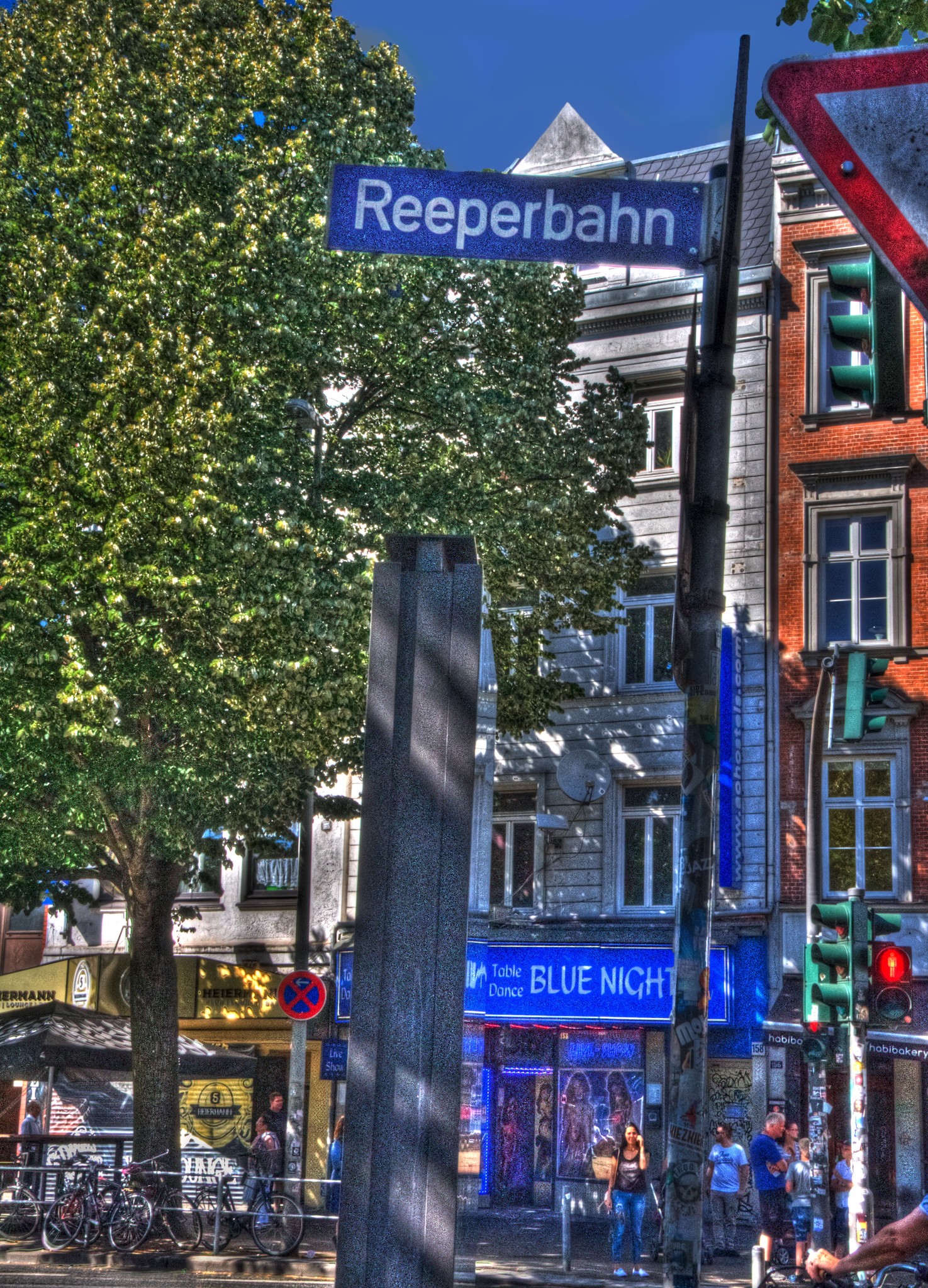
[
  {"xmin": 15, "ymin": 1099, "xmax": 46, "ymax": 1203},
  {"xmin": 319, "ymin": 1115, "xmax": 345, "ymax": 1254},
  {"xmin": 604, "ymin": 1120, "xmax": 652, "ymax": 1279},
  {"xmin": 265, "ymin": 1091, "xmax": 289, "ymax": 1216},
  {"xmin": 784, "ymin": 1136, "xmax": 813, "ymax": 1281},
  {"xmin": 492, "ymin": 1071, "xmax": 635, "ymax": 1196},
  {"xmin": 705, "ymin": 1121, "xmax": 751, "ymax": 1258},
  {"xmin": 773, "ymin": 1120, "xmax": 803, "ymax": 1170},
  {"xmin": 247, "ymin": 1114, "xmax": 283, "ymax": 1231},
  {"xmin": 829, "ymin": 1138, "xmax": 853, "ymax": 1257},
  {"xmin": 804, "ymin": 1192, "xmax": 927, "ymax": 1287},
  {"xmin": 747, "ymin": 1111, "xmax": 791, "ymax": 1282}
]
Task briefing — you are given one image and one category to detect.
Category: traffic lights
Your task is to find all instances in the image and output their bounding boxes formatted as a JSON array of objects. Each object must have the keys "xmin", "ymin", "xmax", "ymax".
[
  {"xmin": 844, "ymin": 650, "xmax": 892, "ymax": 743},
  {"xmin": 867, "ymin": 907, "xmax": 913, "ymax": 1027},
  {"xmin": 827, "ymin": 252, "xmax": 905, "ymax": 419},
  {"xmin": 803, "ymin": 901, "xmax": 854, "ymax": 1026}
]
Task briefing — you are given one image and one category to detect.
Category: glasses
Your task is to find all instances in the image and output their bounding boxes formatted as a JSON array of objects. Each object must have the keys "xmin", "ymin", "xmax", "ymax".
[
  {"xmin": 787, "ymin": 1128, "xmax": 798, "ymax": 1131},
  {"xmin": 841, "ymin": 1148, "xmax": 851, "ymax": 1151}
]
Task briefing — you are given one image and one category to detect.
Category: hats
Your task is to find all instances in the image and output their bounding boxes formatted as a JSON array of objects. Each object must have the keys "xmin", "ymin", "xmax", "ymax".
[{"xmin": 836, "ymin": 1138, "xmax": 851, "ymax": 1146}]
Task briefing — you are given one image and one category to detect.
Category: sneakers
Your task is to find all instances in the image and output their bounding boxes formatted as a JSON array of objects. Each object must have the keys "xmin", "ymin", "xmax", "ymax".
[
  {"xmin": 614, "ymin": 1268, "xmax": 627, "ymax": 1277},
  {"xmin": 785, "ymin": 1273, "xmax": 802, "ymax": 1284},
  {"xmin": 765, "ymin": 1267, "xmax": 786, "ymax": 1280},
  {"xmin": 633, "ymin": 1267, "xmax": 650, "ymax": 1277}
]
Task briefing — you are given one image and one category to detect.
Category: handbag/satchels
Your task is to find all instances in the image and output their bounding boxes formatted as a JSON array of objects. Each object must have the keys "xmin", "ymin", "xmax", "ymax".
[{"xmin": 604, "ymin": 1190, "xmax": 611, "ymax": 1208}]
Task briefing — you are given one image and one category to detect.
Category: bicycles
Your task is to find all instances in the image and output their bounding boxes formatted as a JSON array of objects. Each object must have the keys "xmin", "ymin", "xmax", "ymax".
[
  {"xmin": 0, "ymin": 1150, "xmax": 305, "ymax": 1258},
  {"xmin": 752, "ymin": 1260, "xmax": 928, "ymax": 1288}
]
